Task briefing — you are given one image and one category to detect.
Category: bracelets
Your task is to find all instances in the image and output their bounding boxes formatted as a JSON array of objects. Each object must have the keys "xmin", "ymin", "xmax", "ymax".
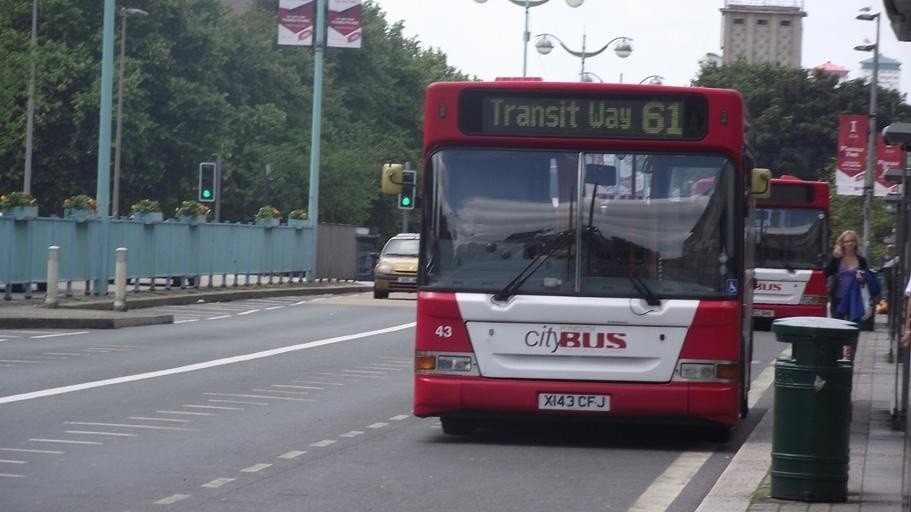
[{"xmin": 904, "ymin": 331, "xmax": 911, "ymax": 333}]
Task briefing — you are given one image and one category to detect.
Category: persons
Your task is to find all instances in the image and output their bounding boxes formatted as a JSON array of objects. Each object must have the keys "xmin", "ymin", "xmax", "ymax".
[
  {"xmin": 827, "ymin": 231, "xmax": 881, "ymax": 359},
  {"xmin": 900, "ymin": 278, "xmax": 911, "ymax": 350}
]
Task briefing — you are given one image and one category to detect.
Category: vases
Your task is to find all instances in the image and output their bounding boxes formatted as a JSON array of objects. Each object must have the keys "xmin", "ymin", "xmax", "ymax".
[
  {"xmin": 287, "ymin": 220, "xmax": 312, "ymax": 230},
  {"xmin": 177, "ymin": 215, "xmax": 207, "ymax": 226},
  {"xmin": 64, "ymin": 209, "xmax": 102, "ymax": 222},
  {"xmin": 131, "ymin": 212, "xmax": 163, "ymax": 225},
  {"xmin": 1, "ymin": 205, "xmax": 38, "ymax": 221},
  {"xmin": 254, "ymin": 219, "xmax": 279, "ymax": 228}
]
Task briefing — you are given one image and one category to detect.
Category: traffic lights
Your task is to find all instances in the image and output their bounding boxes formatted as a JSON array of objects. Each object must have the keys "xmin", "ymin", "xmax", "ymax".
[
  {"xmin": 197, "ymin": 164, "xmax": 213, "ymax": 202},
  {"xmin": 398, "ymin": 169, "xmax": 416, "ymax": 210}
]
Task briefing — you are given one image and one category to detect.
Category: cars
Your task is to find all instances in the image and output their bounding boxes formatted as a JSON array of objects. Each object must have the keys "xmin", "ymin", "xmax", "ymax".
[{"xmin": 373, "ymin": 230, "xmax": 422, "ymax": 300}]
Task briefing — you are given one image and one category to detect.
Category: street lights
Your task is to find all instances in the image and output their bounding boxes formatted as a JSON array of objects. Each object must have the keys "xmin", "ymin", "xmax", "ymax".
[
  {"xmin": 856, "ymin": 0, "xmax": 911, "ymax": 429},
  {"xmin": 472, "ymin": 0, "xmax": 666, "ymax": 197},
  {"xmin": 113, "ymin": 4, "xmax": 150, "ymax": 216}
]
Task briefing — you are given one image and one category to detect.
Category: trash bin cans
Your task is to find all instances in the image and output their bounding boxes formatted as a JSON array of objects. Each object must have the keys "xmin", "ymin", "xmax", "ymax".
[{"xmin": 770, "ymin": 316, "xmax": 860, "ymax": 503}]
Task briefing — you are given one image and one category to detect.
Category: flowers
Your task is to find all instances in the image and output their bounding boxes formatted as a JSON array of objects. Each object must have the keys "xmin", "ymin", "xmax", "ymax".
[
  {"xmin": 175, "ymin": 201, "xmax": 211, "ymax": 217},
  {"xmin": 65, "ymin": 195, "xmax": 99, "ymax": 210},
  {"xmin": 254, "ymin": 205, "xmax": 282, "ymax": 219},
  {"xmin": 131, "ymin": 200, "xmax": 161, "ymax": 212},
  {"xmin": 288, "ymin": 209, "xmax": 309, "ymax": 221},
  {"xmin": 2, "ymin": 192, "xmax": 35, "ymax": 208}
]
